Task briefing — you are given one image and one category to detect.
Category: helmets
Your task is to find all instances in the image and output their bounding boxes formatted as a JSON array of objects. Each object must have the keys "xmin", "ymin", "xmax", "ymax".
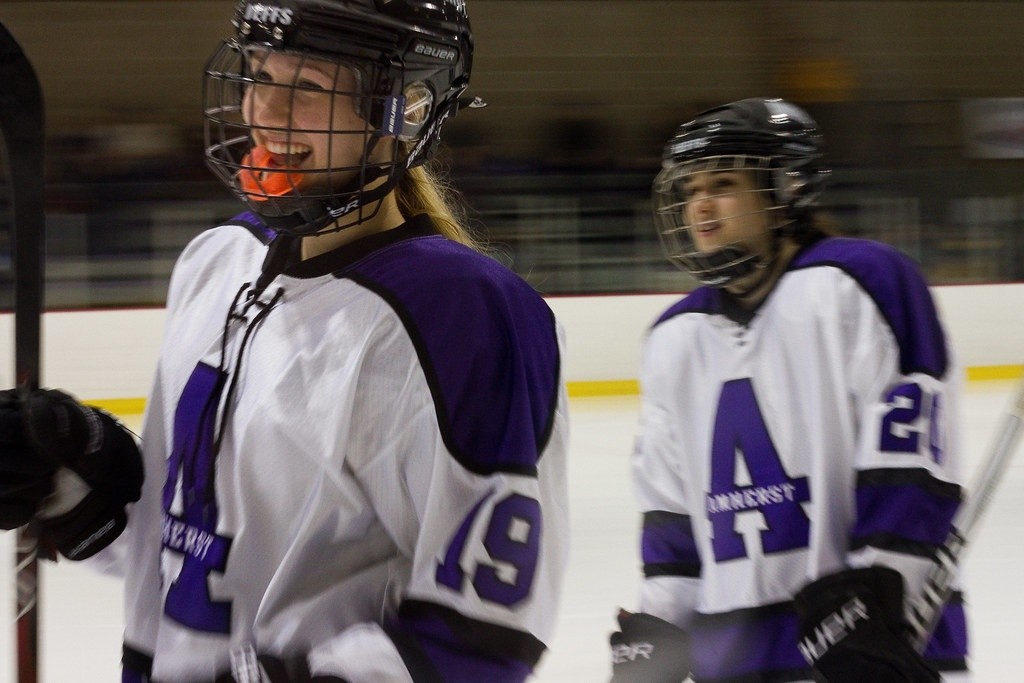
[
  {"xmin": 652, "ymin": 97, "xmax": 830, "ymax": 287},
  {"xmin": 201, "ymin": 1, "xmax": 475, "ymax": 238}
]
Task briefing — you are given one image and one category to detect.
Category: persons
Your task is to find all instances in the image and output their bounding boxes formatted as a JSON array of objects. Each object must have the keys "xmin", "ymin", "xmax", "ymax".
[
  {"xmin": 0, "ymin": 0, "xmax": 567, "ymax": 682},
  {"xmin": 610, "ymin": 97, "xmax": 971, "ymax": 683}
]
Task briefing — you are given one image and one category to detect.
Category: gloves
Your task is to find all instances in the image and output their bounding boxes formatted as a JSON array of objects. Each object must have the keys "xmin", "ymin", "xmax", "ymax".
[
  {"xmin": 787, "ymin": 563, "xmax": 943, "ymax": 683},
  {"xmin": 213, "ymin": 645, "xmax": 314, "ymax": 683},
  {"xmin": 0, "ymin": 384, "xmax": 146, "ymax": 565},
  {"xmin": 610, "ymin": 608, "xmax": 692, "ymax": 683}
]
